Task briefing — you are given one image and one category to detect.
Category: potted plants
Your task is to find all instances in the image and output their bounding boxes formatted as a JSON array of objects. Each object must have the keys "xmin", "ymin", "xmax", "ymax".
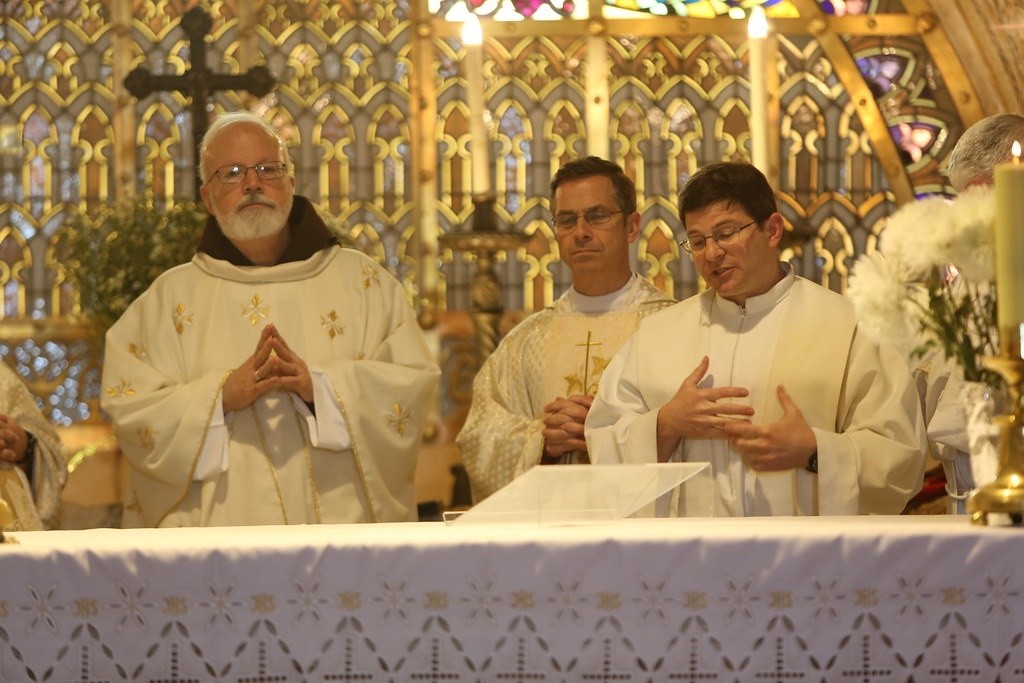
[{"xmin": 42, "ymin": 200, "xmax": 210, "ymax": 525}]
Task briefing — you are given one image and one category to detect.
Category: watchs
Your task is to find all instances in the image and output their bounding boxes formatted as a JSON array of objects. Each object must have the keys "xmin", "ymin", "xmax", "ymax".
[{"xmin": 804, "ymin": 451, "xmax": 818, "ymax": 473}]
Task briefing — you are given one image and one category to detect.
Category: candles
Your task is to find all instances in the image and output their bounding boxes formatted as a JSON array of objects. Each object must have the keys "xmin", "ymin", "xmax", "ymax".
[
  {"xmin": 587, "ymin": 35, "xmax": 611, "ymax": 160},
  {"xmin": 992, "ymin": 140, "xmax": 1024, "ymax": 327},
  {"xmin": 461, "ymin": 0, "xmax": 493, "ymax": 198},
  {"xmin": 745, "ymin": 0, "xmax": 768, "ymax": 180}
]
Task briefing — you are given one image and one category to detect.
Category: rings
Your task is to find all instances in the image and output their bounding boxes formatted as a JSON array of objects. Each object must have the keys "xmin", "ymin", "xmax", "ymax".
[{"xmin": 255, "ymin": 371, "xmax": 261, "ymax": 379}]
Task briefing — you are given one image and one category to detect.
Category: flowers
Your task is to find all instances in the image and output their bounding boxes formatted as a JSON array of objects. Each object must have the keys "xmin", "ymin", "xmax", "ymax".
[{"xmin": 845, "ymin": 182, "xmax": 1000, "ymax": 378}]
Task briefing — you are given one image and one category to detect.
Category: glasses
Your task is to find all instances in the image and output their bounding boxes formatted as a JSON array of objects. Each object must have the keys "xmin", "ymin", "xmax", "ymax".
[
  {"xmin": 551, "ymin": 209, "xmax": 632, "ymax": 228},
  {"xmin": 206, "ymin": 161, "xmax": 286, "ymax": 185},
  {"xmin": 679, "ymin": 210, "xmax": 776, "ymax": 253}
]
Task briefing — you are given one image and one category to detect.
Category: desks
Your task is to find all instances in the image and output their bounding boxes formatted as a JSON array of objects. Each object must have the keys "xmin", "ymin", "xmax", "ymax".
[{"xmin": 0, "ymin": 515, "xmax": 1024, "ymax": 683}]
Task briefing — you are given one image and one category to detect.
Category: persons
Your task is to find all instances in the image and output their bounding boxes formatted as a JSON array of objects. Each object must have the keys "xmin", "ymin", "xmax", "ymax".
[
  {"xmin": 909, "ymin": 112, "xmax": 1024, "ymax": 514},
  {"xmin": 0, "ymin": 357, "xmax": 68, "ymax": 530},
  {"xmin": 584, "ymin": 162, "xmax": 927, "ymax": 519},
  {"xmin": 454, "ymin": 156, "xmax": 678, "ymax": 505},
  {"xmin": 100, "ymin": 112, "xmax": 441, "ymax": 528}
]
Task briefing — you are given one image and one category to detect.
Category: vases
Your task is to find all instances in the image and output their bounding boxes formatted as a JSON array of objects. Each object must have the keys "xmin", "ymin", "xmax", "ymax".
[{"xmin": 960, "ymin": 382, "xmax": 1016, "ymax": 527}]
